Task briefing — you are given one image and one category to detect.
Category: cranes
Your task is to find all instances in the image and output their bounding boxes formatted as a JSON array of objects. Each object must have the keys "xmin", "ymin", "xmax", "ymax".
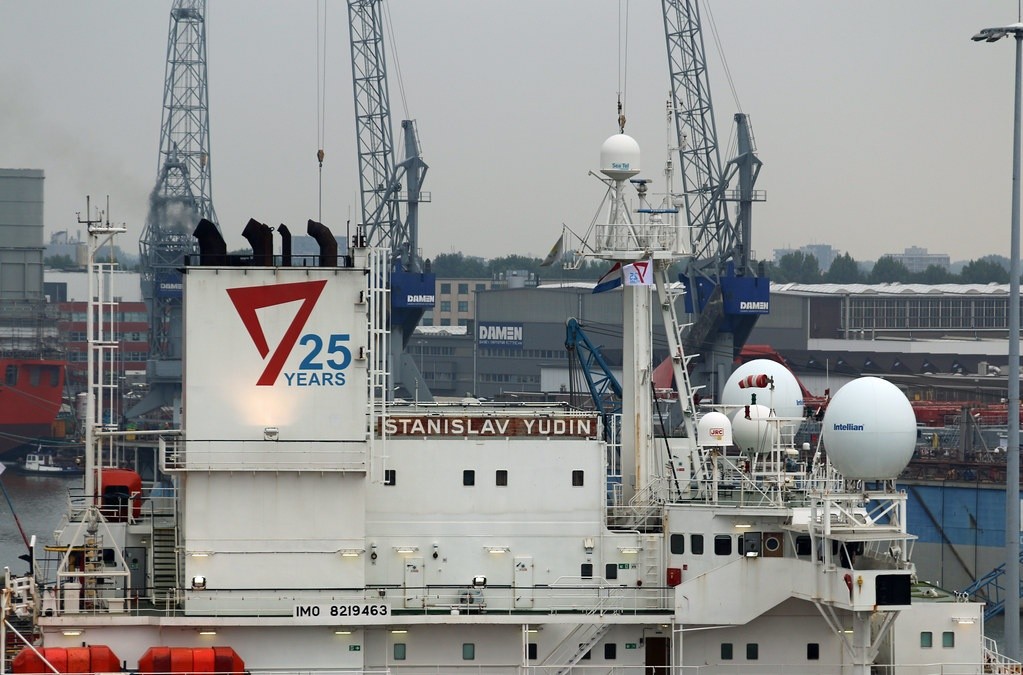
[
  {"xmin": 121, "ymin": 1, "xmax": 221, "ymax": 428},
  {"xmin": 313, "ymin": 0, "xmax": 435, "ymax": 406},
  {"xmin": 616, "ymin": 0, "xmax": 773, "ymax": 409}
]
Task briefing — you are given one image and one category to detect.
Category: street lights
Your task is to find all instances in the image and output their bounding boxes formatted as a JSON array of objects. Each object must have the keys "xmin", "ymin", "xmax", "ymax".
[{"xmin": 968, "ymin": 23, "xmax": 1023, "ymax": 664}]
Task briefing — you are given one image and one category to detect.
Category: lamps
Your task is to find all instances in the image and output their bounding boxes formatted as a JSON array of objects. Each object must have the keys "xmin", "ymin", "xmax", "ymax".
[
  {"xmin": 395, "ymin": 546, "xmax": 418, "ymax": 553},
  {"xmin": 61, "ymin": 629, "xmax": 84, "ymax": 636},
  {"xmin": 617, "ymin": 546, "xmax": 643, "ymax": 554},
  {"xmin": 837, "ymin": 628, "xmax": 854, "ymax": 633},
  {"xmin": 198, "ymin": 628, "xmax": 217, "ymax": 635},
  {"xmin": 473, "ymin": 577, "xmax": 486, "ymax": 589},
  {"xmin": 264, "ymin": 426, "xmax": 279, "ymax": 440},
  {"xmin": 521, "ymin": 625, "xmax": 543, "ymax": 632},
  {"xmin": 342, "ymin": 549, "xmax": 366, "ymax": 556},
  {"xmin": 956, "ymin": 618, "xmax": 976, "ymax": 623},
  {"xmin": 746, "ymin": 550, "xmax": 758, "ymax": 557},
  {"xmin": 485, "ymin": 546, "xmax": 511, "ymax": 554},
  {"xmin": 335, "ymin": 626, "xmax": 353, "ymax": 635},
  {"xmin": 391, "ymin": 626, "xmax": 408, "ymax": 634},
  {"xmin": 733, "ymin": 521, "xmax": 753, "ymax": 527},
  {"xmin": 190, "ymin": 552, "xmax": 209, "ymax": 556},
  {"xmin": 192, "ymin": 576, "xmax": 206, "ymax": 590}
]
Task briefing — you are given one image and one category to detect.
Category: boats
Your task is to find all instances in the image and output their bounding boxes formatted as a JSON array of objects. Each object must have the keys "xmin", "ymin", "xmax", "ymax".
[
  {"xmin": 138, "ymin": 645, "xmax": 246, "ymax": 675},
  {"xmin": 17, "ymin": 441, "xmax": 85, "ymax": 476},
  {"xmin": 0, "ymin": 0, "xmax": 1022, "ymax": 675},
  {"xmin": 94, "ymin": 467, "xmax": 142, "ymax": 523},
  {"xmin": 9, "ymin": 644, "xmax": 121, "ymax": 673}
]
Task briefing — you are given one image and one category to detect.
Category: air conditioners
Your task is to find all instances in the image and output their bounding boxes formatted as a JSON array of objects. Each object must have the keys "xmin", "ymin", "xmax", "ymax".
[{"xmin": 458, "ymin": 590, "xmax": 482, "ymax": 604}]
